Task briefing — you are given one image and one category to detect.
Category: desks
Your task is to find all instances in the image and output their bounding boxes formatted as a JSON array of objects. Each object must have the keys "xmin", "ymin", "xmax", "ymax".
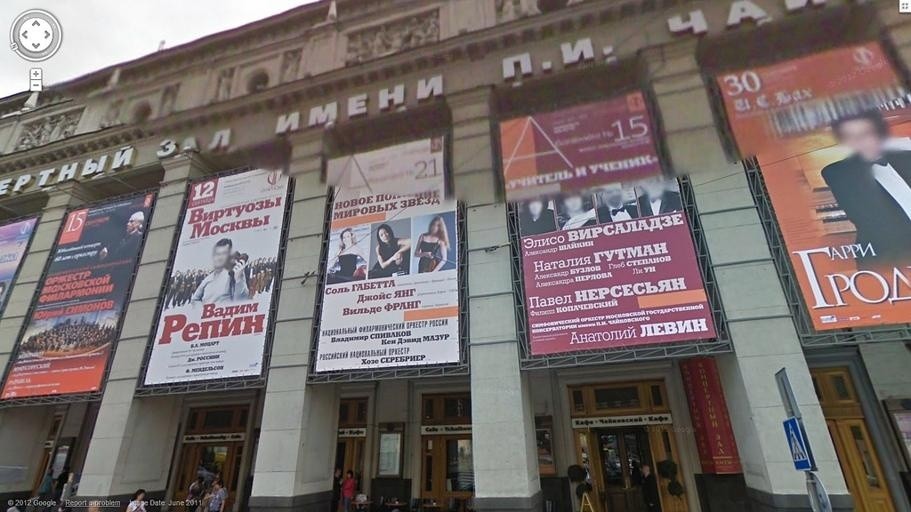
[{"xmin": 350, "ymin": 495, "xmax": 449, "ymax": 512}]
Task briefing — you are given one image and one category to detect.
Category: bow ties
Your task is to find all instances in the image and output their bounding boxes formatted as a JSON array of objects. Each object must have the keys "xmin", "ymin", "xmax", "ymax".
[
  {"xmin": 864, "ymin": 157, "xmax": 889, "ymax": 169},
  {"xmin": 612, "ymin": 207, "xmax": 625, "ymax": 216},
  {"xmin": 650, "ymin": 194, "xmax": 663, "ymax": 203}
]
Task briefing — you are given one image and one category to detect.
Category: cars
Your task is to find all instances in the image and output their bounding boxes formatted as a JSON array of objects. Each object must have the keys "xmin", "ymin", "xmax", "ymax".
[
  {"xmin": 599, "ymin": 455, "xmax": 638, "ymax": 474},
  {"xmin": 196, "ymin": 465, "xmax": 216, "ymax": 480}
]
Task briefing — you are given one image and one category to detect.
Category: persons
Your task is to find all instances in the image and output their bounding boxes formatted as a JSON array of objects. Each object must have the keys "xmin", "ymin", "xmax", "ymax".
[
  {"xmin": 214, "ymin": 460, "xmax": 224, "ymax": 478},
  {"xmin": 414, "ymin": 216, "xmax": 450, "ymax": 273},
  {"xmin": 368, "ymin": 223, "xmax": 411, "ymax": 279},
  {"xmin": 558, "ymin": 191, "xmax": 598, "ymax": 229},
  {"xmin": 520, "ymin": 197, "xmax": 556, "ymax": 236},
  {"xmin": 822, "ymin": 107, "xmax": 911, "ymax": 272},
  {"xmin": 601, "ymin": 436, "xmax": 615, "ymax": 471},
  {"xmin": 339, "ymin": 470, "xmax": 357, "ymax": 511},
  {"xmin": 327, "ymin": 227, "xmax": 367, "ymax": 283},
  {"xmin": 200, "ymin": 445, "xmax": 217, "ymax": 465},
  {"xmin": 598, "ymin": 182, "xmax": 638, "ymax": 223},
  {"xmin": 164, "ymin": 255, "xmax": 277, "ymax": 309},
  {"xmin": 191, "ymin": 236, "xmax": 250, "ymax": 304},
  {"xmin": 333, "ymin": 467, "xmax": 345, "ymax": 512},
  {"xmin": 640, "ymin": 465, "xmax": 660, "ymax": 511},
  {"xmin": 6, "ymin": 469, "xmax": 230, "ymax": 511},
  {"xmin": 97, "ymin": 211, "xmax": 145, "ymax": 264},
  {"xmin": 638, "ymin": 177, "xmax": 683, "ymax": 216}
]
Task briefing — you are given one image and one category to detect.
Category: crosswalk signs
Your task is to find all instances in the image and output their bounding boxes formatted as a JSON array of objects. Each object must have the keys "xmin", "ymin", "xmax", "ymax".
[{"xmin": 782, "ymin": 416, "xmax": 813, "ymax": 471}]
